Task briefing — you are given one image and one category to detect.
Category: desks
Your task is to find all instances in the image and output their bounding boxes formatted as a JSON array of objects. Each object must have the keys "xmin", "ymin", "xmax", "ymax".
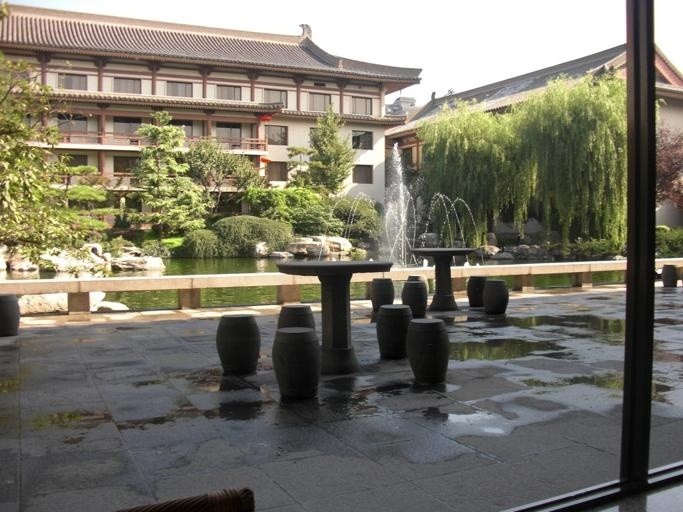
[
  {"xmin": 275, "ymin": 260, "xmax": 393, "ymax": 374},
  {"xmin": 409, "ymin": 247, "xmax": 476, "ymax": 312}
]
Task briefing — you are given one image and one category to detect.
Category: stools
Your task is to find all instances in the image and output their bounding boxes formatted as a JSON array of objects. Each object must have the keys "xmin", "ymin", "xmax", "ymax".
[
  {"xmin": 0, "ymin": 294, "xmax": 19, "ymax": 336},
  {"xmin": 216, "ymin": 275, "xmax": 509, "ymax": 401},
  {"xmin": 661, "ymin": 264, "xmax": 678, "ymax": 287}
]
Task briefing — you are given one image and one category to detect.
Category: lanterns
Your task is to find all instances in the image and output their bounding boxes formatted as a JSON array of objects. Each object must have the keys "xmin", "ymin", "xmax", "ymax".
[
  {"xmin": 260, "ymin": 156, "xmax": 272, "ymax": 166},
  {"xmin": 260, "ymin": 113, "xmax": 273, "ymax": 126}
]
